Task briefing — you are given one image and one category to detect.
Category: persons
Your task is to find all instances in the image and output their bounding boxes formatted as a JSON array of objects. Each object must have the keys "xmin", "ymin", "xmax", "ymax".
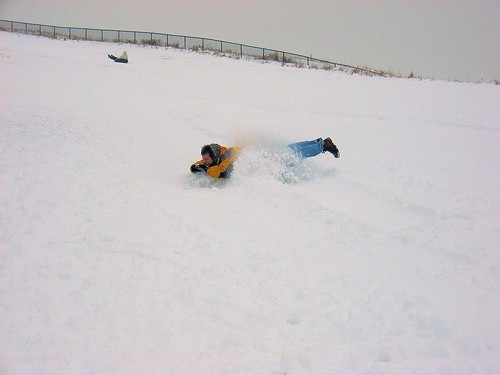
[
  {"xmin": 190, "ymin": 137, "xmax": 340, "ymax": 179},
  {"xmin": 108, "ymin": 51, "xmax": 128, "ymax": 60}
]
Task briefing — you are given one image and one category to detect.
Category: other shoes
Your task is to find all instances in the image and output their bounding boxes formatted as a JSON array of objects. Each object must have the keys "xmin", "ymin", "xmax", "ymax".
[
  {"xmin": 316, "ymin": 138, "xmax": 323, "ymax": 143},
  {"xmin": 322, "ymin": 137, "xmax": 340, "ymax": 158}
]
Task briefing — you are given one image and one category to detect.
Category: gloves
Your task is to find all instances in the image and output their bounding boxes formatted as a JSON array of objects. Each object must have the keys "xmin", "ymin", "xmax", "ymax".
[{"xmin": 194, "ymin": 164, "xmax": 209, "ymax": 174}]
